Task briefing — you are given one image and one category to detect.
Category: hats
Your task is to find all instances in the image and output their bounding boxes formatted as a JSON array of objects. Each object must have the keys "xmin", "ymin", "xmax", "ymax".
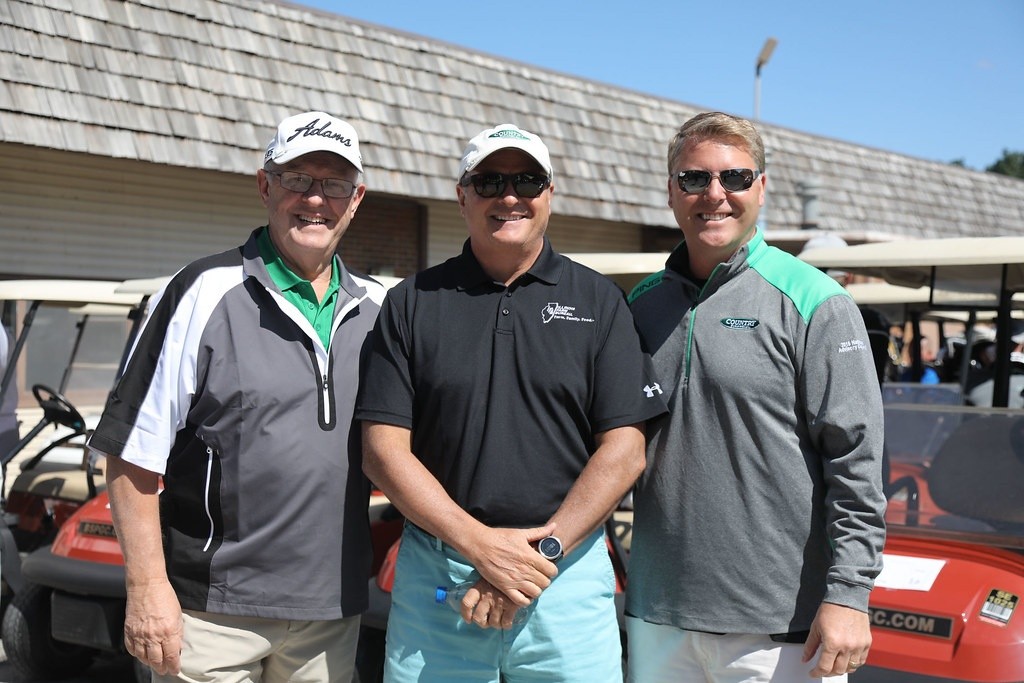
[
  {"xmin": 263, "ymin": 111, "xmax": 364, "ymax": 175},
  {"xmin": 458, "ymin": 124, "xmax": 553, "ymax": 183}
]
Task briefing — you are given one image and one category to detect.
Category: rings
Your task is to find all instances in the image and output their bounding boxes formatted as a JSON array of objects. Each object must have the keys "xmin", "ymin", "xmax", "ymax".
[
  {"xmin": 504, "ymin": 623, "xmax": 512, "ymax": 627},
  {"xmin": 849, "ymin": 662, "xmax": 860, "ymax": 668}
]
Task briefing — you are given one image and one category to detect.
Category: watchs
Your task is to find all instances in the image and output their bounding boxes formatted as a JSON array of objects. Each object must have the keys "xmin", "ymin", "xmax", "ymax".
[{"xmin": 537, "ymin": 536, "xmax": 564, "ymax": 562}]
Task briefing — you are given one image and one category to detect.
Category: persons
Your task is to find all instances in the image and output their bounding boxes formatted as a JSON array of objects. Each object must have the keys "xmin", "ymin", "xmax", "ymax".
[
  {"xmin": 86, "ymin": 112, "xmax": 388, "ymax": 683},
  {"xmin": 626, "ymin": 113, "xmax": 884, "ymax": 683},
  {"xmin": 358, "ymin": 124, "xmax": 670, "ymax": 683},
  {"xmin": 800, "ymin": 235, "xmax": 892, "ymax": 383}
]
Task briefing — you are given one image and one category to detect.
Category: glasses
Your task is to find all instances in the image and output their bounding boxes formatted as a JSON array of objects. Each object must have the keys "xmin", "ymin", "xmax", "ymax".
[
  {"xmin": 671, "ymin": 168, "xmax": 762, "ymax": 193},
  {"xmin": 461, "ymin": 172, "xmax": 552, "ymax": 198},
  {"xmin": 262, "ymin": 168, "xmax": 359, "ymax": 199}
]
